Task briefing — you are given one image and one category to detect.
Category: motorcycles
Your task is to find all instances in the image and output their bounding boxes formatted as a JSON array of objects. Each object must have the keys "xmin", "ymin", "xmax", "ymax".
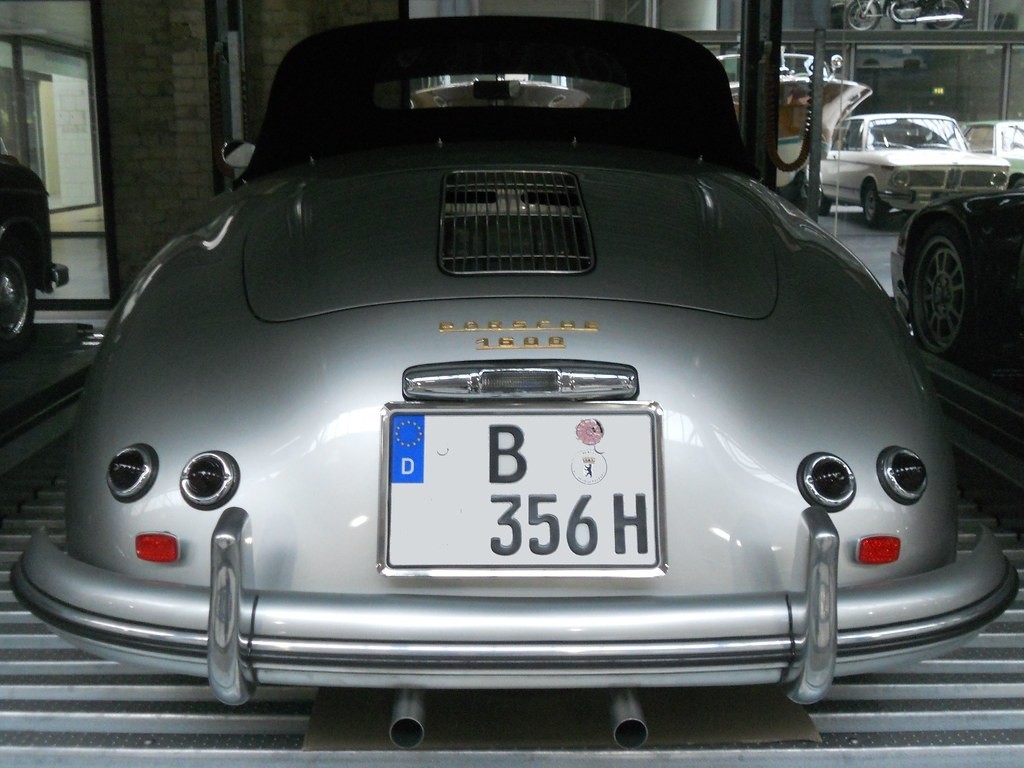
[{"xmin": 843, "ymin": 0, "xmax": 972, "ymax": 31}]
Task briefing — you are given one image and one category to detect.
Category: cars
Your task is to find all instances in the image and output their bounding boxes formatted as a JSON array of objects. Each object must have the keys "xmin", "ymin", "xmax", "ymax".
[
  {"xmin": 960, "ymin": 120, "xmax": 1024, "ymax": 178},
  {"xmin": 0, "ymin": 140, "xmax": 70, "ymax": 362},
  {"xmin": 890, "ymin": 173, "xmax": 1024, "ymax": 384},
  {"xmin": 710, "ymin": 45, "xmax": 872, "ymax": 203},
  {"xmin": 7, "ymin": 17, "xmax": 1017, "ymax": 753},
  {"xmin": 817, "ymin": 112, "xmax": 1010, "ymax": 230}
]
